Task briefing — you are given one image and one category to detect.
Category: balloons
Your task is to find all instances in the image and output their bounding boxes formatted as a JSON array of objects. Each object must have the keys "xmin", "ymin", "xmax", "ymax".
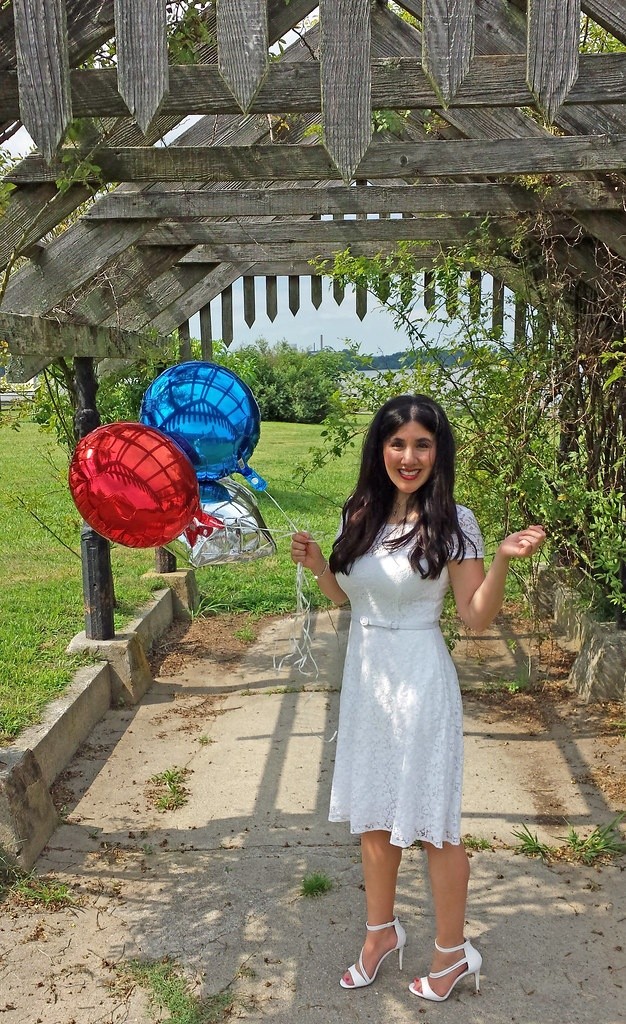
[{"xmin": 67, "ymin": 359, "xmax": 278, "ymax": 568}]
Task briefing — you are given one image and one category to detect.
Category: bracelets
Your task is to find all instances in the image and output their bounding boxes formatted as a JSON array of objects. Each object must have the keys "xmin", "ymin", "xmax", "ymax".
[{"xmin": 312, "ymin": 560, "xmax": 330, "ymax": 579}]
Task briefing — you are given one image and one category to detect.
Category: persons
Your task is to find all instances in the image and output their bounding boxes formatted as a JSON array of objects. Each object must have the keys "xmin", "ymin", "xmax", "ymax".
[{"xmin": 291, "ymin": 394, "xmax": 547, "ymax": 1002}]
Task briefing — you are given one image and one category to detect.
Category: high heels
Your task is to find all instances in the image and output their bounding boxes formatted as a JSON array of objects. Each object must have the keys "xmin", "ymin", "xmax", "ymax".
[
  {"xmin": 339, "ymin": 916, "xmax": 406, "ymax": 988},
  {"xmin": 409, "ymin": 937, "xmax": 482, "ymax": 1001}
]
